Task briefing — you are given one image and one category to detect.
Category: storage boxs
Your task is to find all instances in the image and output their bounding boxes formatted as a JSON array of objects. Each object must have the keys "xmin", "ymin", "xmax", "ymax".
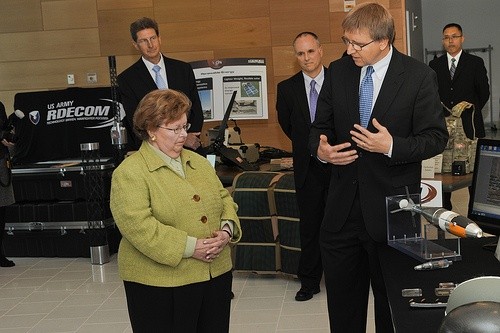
[{"xmin": 4, "ymin": 157, "xmax": 122, "ymax": 261}]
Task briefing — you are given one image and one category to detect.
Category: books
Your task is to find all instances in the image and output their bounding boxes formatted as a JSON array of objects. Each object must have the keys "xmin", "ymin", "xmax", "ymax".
[{"xmin": 271, "ymin": 158, "xmax": 295, "ymax": 172}]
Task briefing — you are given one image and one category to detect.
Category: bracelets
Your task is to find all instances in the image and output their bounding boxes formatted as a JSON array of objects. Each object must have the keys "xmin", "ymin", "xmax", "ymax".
[{"xmin": 222, "ymin": 229, "xmax": 232, "ymax": 242}]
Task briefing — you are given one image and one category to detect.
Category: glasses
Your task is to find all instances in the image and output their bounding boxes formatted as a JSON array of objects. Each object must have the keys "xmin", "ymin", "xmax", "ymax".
[
  {"xmin": 444, "ymin": 34, "xmax": 462, "ymax": 41},
  {"xmin": 341, "ymin": 36, "xmax": 381, "ymax": 51},
  {"xmin": 157, "ymin": 123, "xmax": 193, "ymax": 134}
]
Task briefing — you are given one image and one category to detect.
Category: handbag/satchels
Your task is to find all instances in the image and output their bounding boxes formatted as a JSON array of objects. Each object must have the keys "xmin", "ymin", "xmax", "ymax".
[{"xmin": 5, "ymin": 113, "xmax": 21, "ymax": 143}]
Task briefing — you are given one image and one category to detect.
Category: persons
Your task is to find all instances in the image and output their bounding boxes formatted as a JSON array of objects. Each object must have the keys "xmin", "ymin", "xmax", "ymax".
[
  {"xmin": 112, "ymin": 18, "xmax": 204, "ymax": 176},
  {"xmin": 276, "ymin": 31, "xmax": 354, "ymax": 301},
  {"xmin": 0, "ymin": 100, "xmax": 19, "ymax": 268},
  {"xmin": 427, "ymin": 23, "xmax": 490, "ymax": 154},
  {"xmin": 307, "ymin": 4, "xmax": 447, "ymax": 332},
  {"xmin": 110, "ymin": 88, "xmax": 244, "ymax": 333}
]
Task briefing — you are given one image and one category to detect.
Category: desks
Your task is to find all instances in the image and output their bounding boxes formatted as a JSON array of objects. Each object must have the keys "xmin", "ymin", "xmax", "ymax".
[
  {"xmin": 422, "ymin": 170, "xmax": 473, "ymax": 213},
  {"xmin": 375, "ymin": 236, "xmax": 500, "ymax": 333}
]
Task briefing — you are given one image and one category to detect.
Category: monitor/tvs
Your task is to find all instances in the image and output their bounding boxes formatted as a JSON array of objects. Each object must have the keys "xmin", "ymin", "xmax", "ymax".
[{"xmin": 467, "ymin": 139, "xmax": 500, "ymax": 250}]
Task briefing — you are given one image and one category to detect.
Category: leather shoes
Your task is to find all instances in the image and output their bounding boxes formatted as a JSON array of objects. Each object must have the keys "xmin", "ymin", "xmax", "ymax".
[
  {"xmin": 0, "ymin": 257, "xmax": 15, "ymax": 267},
  {"xmin": 295, "ymin": 284, "xmax": 320, "ymax": 301}
]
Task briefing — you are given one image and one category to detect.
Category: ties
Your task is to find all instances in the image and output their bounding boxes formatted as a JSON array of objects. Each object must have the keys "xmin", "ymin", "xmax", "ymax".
[
  {"xmin": 153, "ymin": 65, "xmax": 167, "ymax": 89},
  {"xmin": 449, "ymin": 58, "xmax": 456, "ymax": 81},
  {"xmin": 358, "ymin": 66, "xmax": 374, "ymax": 130},
  {"xmin": 308, "ymin": 81, "xmax": 318, "ymax": 124}
]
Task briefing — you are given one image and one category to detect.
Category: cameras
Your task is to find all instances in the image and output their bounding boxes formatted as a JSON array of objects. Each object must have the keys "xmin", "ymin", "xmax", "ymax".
[{"xmin": 0, "ymin": 110, "xmax": 25, "ymax": 142}]
